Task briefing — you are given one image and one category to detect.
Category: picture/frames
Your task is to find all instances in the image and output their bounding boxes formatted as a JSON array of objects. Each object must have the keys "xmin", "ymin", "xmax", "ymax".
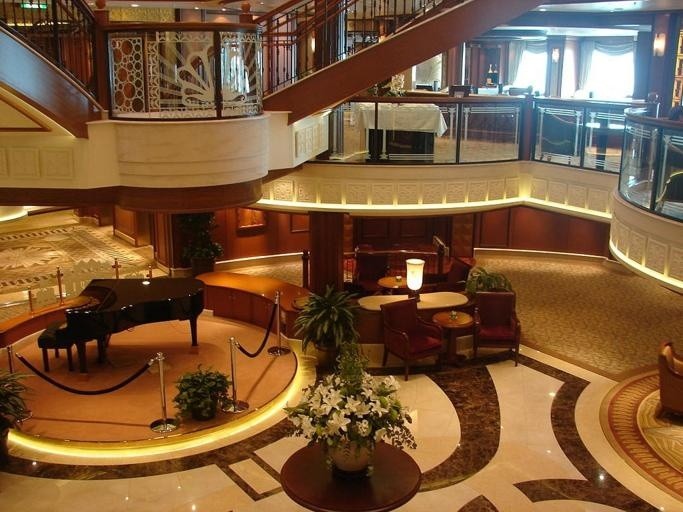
[{"xmin": 236, "ymin": 208, "xmax": 268, "ymax": 232}]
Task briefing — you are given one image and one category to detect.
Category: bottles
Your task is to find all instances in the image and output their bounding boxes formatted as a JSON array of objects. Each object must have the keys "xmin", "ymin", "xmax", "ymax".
[
  {"xmin": 487, "ymin": 63, "xmax": 492, "ymax": 84},
  {"xmin": 494, "ymin": 64, "xmax": 499, "ymax": 84}
]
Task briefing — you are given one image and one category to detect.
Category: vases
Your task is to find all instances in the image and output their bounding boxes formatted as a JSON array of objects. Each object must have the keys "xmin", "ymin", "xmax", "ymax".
[{"xmin": 328, "ymin": 439, "xmax": 375, "ymax": 473}]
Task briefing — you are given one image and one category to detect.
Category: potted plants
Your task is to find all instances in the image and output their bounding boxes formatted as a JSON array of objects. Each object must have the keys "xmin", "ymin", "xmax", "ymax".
[
  {"xmin": 290, "ymin": 283, "xmax": 360, "ymax": 367},
  {"xmin": 183, "ymin": 229, "xmax": 223, "ymax": 276},
  {"xmin": 170, "ymin": 362, "xmax": 234, "ymax": 424},
  {"xmin": 0, "ymin": 370, "xmax": 30, "ymax": 474}
]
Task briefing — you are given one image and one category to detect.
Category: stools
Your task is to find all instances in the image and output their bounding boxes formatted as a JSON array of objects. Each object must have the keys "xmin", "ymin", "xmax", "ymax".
[{"xmin": 37, "ymin": 319, "xmax": 78, "ymax": 373}]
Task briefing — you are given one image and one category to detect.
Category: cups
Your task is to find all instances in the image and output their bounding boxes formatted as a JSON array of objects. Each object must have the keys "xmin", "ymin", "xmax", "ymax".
[
  {"xmin": 451, "ymin": 311, "xmax": 457, "ymax": 320},
  {"xmin": 396, "ymin": 275, "xmax": 402, "ymax": 282}
]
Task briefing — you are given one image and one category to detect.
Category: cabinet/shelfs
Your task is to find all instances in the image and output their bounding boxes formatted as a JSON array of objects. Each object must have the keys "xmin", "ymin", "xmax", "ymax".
[
  {"xmin": 212, "ymin": 288, "xmax": 251, "ymax": 322},
  {"xmin": 252, "ymin": 295, "xmax": 277, "ymax": 334}
]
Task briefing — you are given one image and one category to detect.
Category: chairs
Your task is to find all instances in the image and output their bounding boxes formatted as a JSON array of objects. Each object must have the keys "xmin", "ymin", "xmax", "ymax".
[
  {"xmin": 424, "ymin": 257, "xmax": 471, "ymax": 292},
  {"xmin": 469, "ymin": 290, "xmax": 523, "ymax": 368},
  {"xmin": 381, "ymin": 298, "xmax": 447, "ymax": 382},
  {"xmin": 655, "ymin": 337, "xmax": 682, "ymax": 424},
  {"xmin": 536, "ymin": 107, "xmax": 576, "ymax": 166}
]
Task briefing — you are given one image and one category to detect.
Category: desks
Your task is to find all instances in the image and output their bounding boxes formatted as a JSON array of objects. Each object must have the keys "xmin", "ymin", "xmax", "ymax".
[
  {"xmin": 432, "ymin": 310, "xmax": 475, "ymax": 370},
  {"xmin": 581, "ymin": 120, "xmax": 628, "ymax": 174},
  {"xmin": 278, "ymin": 440, "xmax": 423, "ymax": 512},
  {"xmin": 377, "ymin": 275, "xmax": 410, "ymax": 295},
  {"xmin": 356, "ymin": 292, "xmax": 471, "ymax": 313}
]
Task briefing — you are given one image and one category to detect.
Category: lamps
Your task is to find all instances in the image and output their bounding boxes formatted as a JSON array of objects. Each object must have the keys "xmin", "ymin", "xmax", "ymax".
[
  {"xmin": 652, "ymin": 30, "xmax": 666, "ymax": 59},
  {"xmin": 404, "ymin": 257, "xmax": 425, "ymax": 303}
]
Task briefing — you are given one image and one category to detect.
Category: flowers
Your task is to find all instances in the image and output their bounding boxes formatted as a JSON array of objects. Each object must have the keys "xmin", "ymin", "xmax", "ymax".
[{"xmin": 279, "ymin": 340, "xmax": 420, "ymax": 482}]
